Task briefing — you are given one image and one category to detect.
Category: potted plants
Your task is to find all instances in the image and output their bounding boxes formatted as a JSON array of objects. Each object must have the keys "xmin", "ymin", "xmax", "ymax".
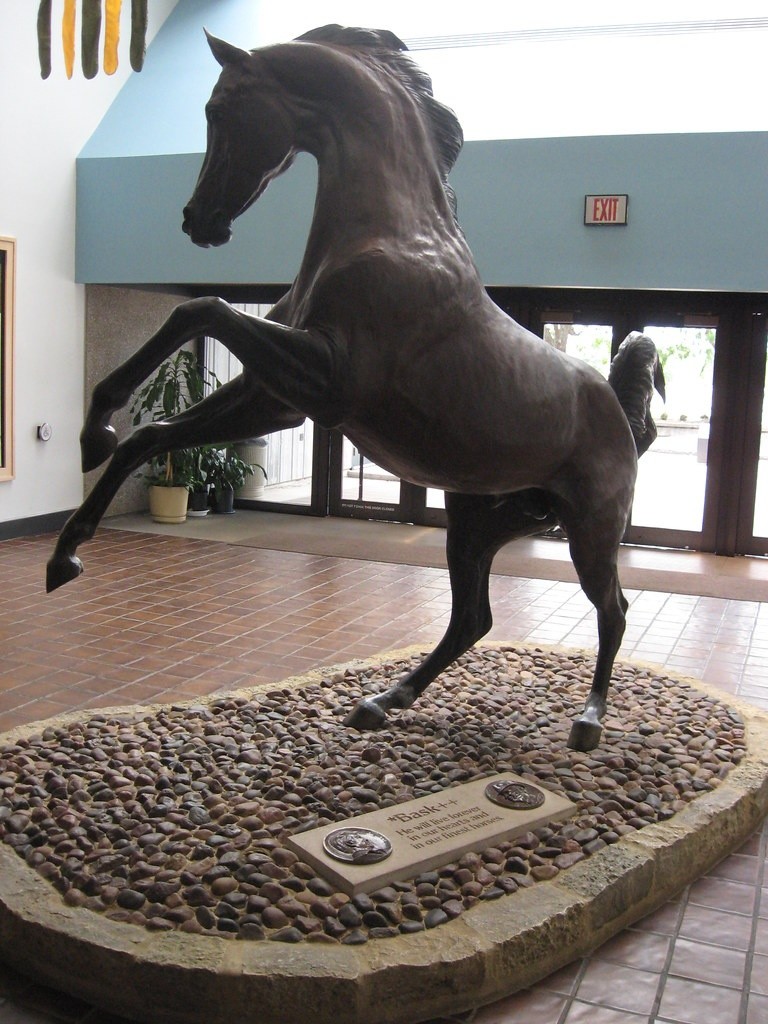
[{"xmin": 123, "ymin": 347, "xmax": 270, "ymax": 523}]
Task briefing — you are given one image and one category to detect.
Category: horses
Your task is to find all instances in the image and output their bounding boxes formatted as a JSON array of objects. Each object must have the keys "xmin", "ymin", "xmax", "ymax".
[{"xmin": 44, "ymin": 22, "xmax": 668, "ymax": 750}]
